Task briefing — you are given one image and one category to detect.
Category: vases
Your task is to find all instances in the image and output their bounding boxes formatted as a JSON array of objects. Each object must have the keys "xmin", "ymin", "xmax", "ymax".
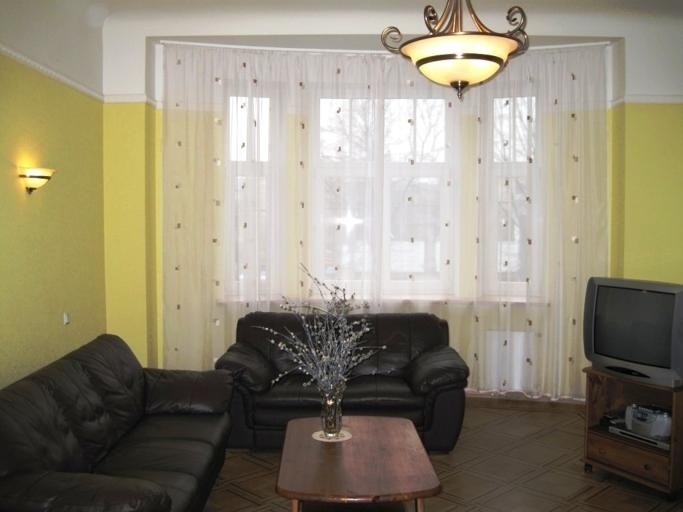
[{"xmin": 317, "ymin": 385, "xmax": 346, "ymax": 439}]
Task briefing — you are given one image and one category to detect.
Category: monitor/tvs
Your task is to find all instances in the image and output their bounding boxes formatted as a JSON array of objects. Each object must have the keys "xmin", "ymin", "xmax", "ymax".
[{"xmin": 584, "ymin": 277, "xmax": 683, "ymax": 393}]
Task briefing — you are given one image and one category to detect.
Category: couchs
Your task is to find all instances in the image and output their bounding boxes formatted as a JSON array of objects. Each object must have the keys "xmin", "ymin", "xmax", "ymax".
[
  {"xmin": 215, "ymin": 311, "xmax": 469, "ymax": 455},
  {"xmin": 0, "ymin": 334, "xmax": 236, "ymax": 512}
]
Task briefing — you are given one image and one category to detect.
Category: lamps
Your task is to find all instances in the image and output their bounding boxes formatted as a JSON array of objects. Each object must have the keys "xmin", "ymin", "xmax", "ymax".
[
  {"xmin": 380, "ymin": 0, "xmax": 529, "ymax": 99},
  {"xmin": 19, "ymin": 167, "xmax": 56, "ymax": 196}
]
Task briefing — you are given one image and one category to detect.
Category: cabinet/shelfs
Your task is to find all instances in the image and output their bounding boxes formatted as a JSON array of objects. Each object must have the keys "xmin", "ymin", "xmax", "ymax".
[{"xmin": 579, "ymin": 368, "xmax": 683, "ymax": 500}]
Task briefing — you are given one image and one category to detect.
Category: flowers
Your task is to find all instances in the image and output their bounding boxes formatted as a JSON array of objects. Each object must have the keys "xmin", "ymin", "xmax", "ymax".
[{"xmin": 252, "ymin": 263, "xmax": 389, "ymax": 429}]
